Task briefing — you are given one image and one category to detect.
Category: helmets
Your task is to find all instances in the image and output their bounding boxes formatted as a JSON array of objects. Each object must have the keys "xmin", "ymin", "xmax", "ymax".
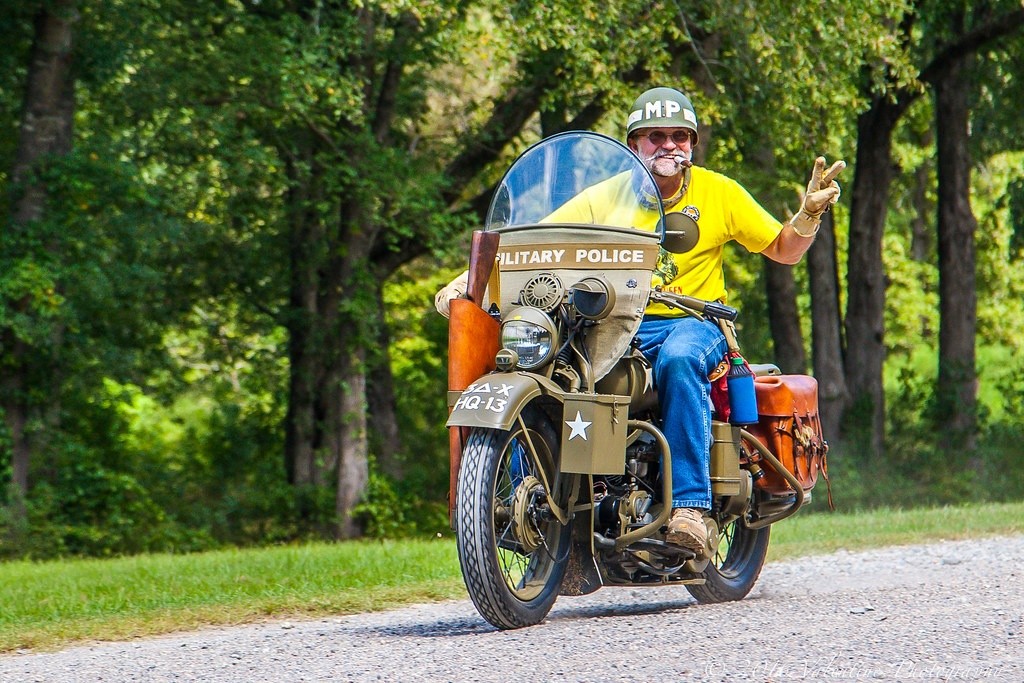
[{"xmin": 626, "ymin": 87, "xmax": 699, "ymax": 147}]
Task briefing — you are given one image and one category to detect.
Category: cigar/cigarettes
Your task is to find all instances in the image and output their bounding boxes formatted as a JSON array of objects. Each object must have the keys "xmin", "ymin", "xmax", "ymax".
[{"xmin": 675, "ymin": 156, "xmax": 693, "ymax": 169}]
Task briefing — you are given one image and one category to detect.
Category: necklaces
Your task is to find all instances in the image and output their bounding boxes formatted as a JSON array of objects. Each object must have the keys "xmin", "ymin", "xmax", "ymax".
[{"xmin": 632, "ymin": 165, "xmax": 691, "ymax": 209}]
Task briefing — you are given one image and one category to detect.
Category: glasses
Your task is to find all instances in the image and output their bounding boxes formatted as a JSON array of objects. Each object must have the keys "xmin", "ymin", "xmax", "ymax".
[{"xmin": 635, "ymin": 130, "xmax": 694, "ymax": 147}]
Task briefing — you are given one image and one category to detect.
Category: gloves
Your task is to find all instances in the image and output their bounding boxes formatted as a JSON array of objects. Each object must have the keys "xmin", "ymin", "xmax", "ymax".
[
  {"xmin": 434, "ymin": 271, "xmax": 468, "ymax": 319},
  {"xmin": 790, "ymin": 155, "xmax": 847, "ymax": 238}
]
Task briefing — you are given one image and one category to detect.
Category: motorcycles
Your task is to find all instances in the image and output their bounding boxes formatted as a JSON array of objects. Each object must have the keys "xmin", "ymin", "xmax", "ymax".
[{"xmin": 436, "ymin": 130, "xmax": 827, "ymax": 628}]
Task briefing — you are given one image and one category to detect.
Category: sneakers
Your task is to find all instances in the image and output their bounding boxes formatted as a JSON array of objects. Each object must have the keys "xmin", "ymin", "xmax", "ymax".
[{"xmin": 666, "ymin": 508, "xmax": 707, "ymax": 553}]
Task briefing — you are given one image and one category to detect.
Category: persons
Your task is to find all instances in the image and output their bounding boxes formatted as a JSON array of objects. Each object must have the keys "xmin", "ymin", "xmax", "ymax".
[{"xmin": 435, "ymin": 88, "xmax": 847, "ymax": 555}]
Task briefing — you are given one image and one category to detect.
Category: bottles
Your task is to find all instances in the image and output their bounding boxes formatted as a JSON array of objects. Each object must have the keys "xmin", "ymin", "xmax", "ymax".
[{"xmin": 728, "ymin": 358, "xmax": 750, "ymax": 375}]
